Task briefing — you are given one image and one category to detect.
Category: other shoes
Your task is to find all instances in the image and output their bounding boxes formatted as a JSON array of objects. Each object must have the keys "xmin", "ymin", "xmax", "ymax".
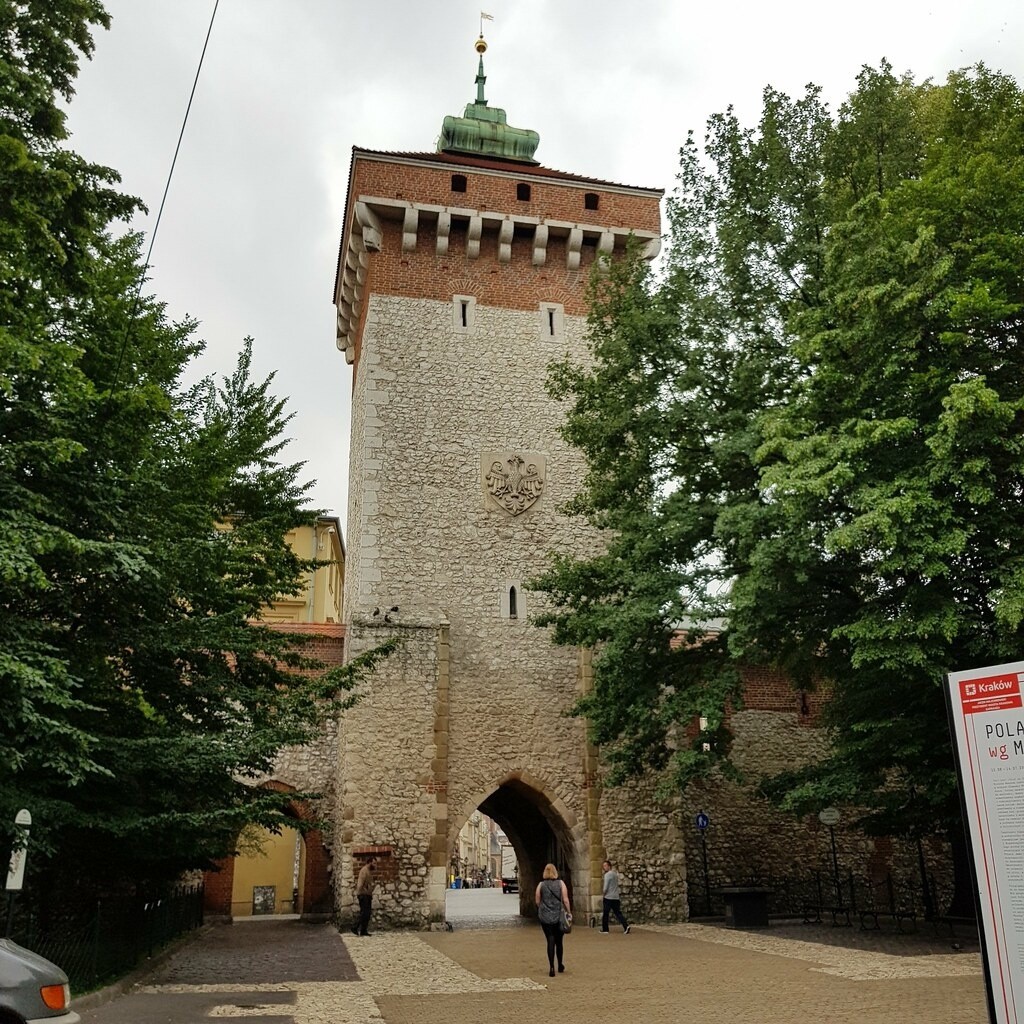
[
  {"xmin": 624, "ymin": 926, "xmax": 630, "ymax": 934},
  {"xmin": 598, "ymin": 930, "xmax": 609, "ymax": 934},
  {"xmin": 360, "ymin": 931, "xmax": 372, "ymax": 936},
  {"xmin": 549, "ymin": 968, "xmax": 555, "ymax": 977},
  {"xmin": 351, "ymin": 927, "xmax": 359, "ymax": 936},
  {"xmin": 558, "ymin": 965, "xmax": 564, "ymax": 972}
]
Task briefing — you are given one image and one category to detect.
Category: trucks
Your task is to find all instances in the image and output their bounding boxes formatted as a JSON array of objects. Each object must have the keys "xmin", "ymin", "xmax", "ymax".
[{"xmin": 500, "ymin": 844, "xmax": 520, "ymax": 894}]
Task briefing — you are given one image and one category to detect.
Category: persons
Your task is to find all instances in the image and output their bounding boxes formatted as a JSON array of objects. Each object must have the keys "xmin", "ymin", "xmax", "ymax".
[
  {"xmin": 351, "ymin": 857, "xmax": 378, "ymax": 936},
  {"xmin": 599, "ymin": 861, "xmax": 630, "ymax": 935},
  {"xmin": 535, "ymin": 864, "xmax": 572, "ymax": 977}
]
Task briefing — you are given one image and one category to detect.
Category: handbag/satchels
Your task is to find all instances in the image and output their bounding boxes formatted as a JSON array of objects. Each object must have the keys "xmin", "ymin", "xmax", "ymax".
[{"xmin": 559, "ymin": 908, "xmax": 571, "ymax": 933}]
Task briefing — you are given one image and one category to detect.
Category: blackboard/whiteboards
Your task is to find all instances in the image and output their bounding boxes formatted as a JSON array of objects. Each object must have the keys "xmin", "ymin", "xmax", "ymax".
[{"xmin": 251, "ymin": 885, "xmax": 277, "ymax": 915}]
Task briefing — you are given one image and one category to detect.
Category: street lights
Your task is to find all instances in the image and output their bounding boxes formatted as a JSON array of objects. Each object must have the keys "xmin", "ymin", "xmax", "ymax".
[{"xmin": 464, "ymin": 857, "xmax": 468, "ymax": 878}]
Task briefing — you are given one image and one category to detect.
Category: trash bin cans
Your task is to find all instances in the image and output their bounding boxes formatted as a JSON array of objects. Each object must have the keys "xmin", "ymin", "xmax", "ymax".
[
  {"xmin": 710, "ymin": 885, "xmax": 776, "ymax": 929},
  {"xmin": 293, "ymin": 888, "xmax": 299, "ymax": 914},
  {"xmin": 455, "ymin": 878, "xmax": 461, "ymax": 889}
]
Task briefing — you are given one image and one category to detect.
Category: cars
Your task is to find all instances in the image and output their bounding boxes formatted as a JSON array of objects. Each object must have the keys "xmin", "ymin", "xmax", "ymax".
[
  {"xmin": 487, "ymin": 879, "xmax": 501, "ymax": 888},
  {"xmin": 0, "ymin": 938, "xmax": 81, "ymax": 1024}
]
